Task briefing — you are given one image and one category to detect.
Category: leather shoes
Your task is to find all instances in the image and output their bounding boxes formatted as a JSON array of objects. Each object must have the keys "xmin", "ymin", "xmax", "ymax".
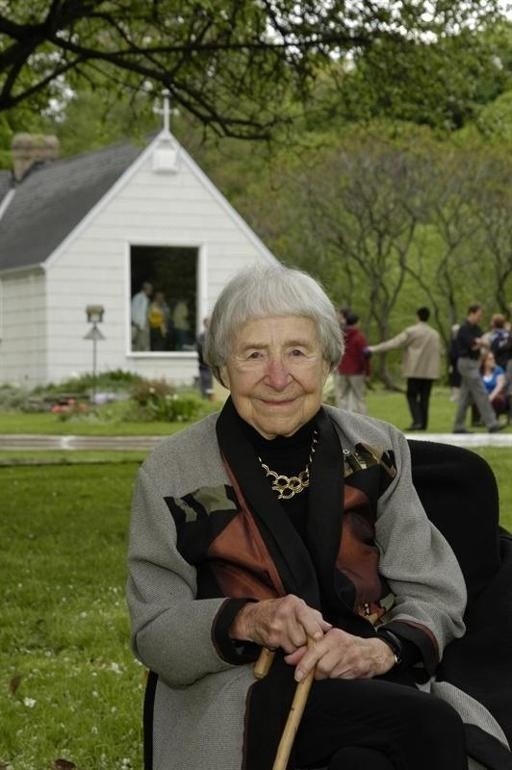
[{"xmin": 453, "ymin": 419, "xmax": 512, "ymax": 433}]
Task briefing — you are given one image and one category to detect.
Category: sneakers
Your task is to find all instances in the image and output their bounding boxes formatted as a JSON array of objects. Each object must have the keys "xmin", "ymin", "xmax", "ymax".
[{"xmin": 407, "ymin": 425, "xmax": 426, "ymax": 430}]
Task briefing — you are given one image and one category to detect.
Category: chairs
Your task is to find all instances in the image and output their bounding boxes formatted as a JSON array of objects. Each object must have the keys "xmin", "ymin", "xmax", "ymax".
[{"xmin": 144, "ymin": 439, "xmax": 512, "ymax": 770}]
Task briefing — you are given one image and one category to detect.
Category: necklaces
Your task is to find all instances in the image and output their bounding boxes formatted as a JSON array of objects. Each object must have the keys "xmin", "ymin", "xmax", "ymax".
[{"xmin": 255, "ymin": 426, "xmax": 318, "ymax": 503}]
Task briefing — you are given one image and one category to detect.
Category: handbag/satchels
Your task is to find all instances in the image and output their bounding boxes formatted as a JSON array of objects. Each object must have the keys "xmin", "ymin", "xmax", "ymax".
[{"xmin": 446, "ymin": 370, "xmax": 462, "ymax": 387}]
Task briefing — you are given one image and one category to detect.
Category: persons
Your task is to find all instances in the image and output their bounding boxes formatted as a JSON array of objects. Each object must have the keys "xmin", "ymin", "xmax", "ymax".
[
  {"xmin": 149, "ymin": 291, "xmax": 174, "ymax": 350},
  {"xmin": 131, "ymin": 282, "xmax": 154, "ymax": 352},
  {"xmin": 124, "ymin": 260, "xmax": 512, "ymax": 769},
  {"xmin": 445, "ymin": 304, "xmax": 511, "ymax": 433},
  {"xmin": 336, "ymin": 314, "xmax": 371, "ymax": 415},
  {"xmin": 338, "ymin": 307, "xmax": 352, "ymax": 328},
  {"xmin": 171, "ymin": 294, "xmax": 197, "ymax": 352},
  {"xmin": 361, "ymin": 306, "xmax": 444, "ymax": 430}
]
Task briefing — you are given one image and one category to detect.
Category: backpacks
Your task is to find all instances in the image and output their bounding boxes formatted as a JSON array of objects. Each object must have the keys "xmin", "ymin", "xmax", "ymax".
[{"xmin": 493, "ymin": 330, "xmax": 509, "ymax": 352}]
